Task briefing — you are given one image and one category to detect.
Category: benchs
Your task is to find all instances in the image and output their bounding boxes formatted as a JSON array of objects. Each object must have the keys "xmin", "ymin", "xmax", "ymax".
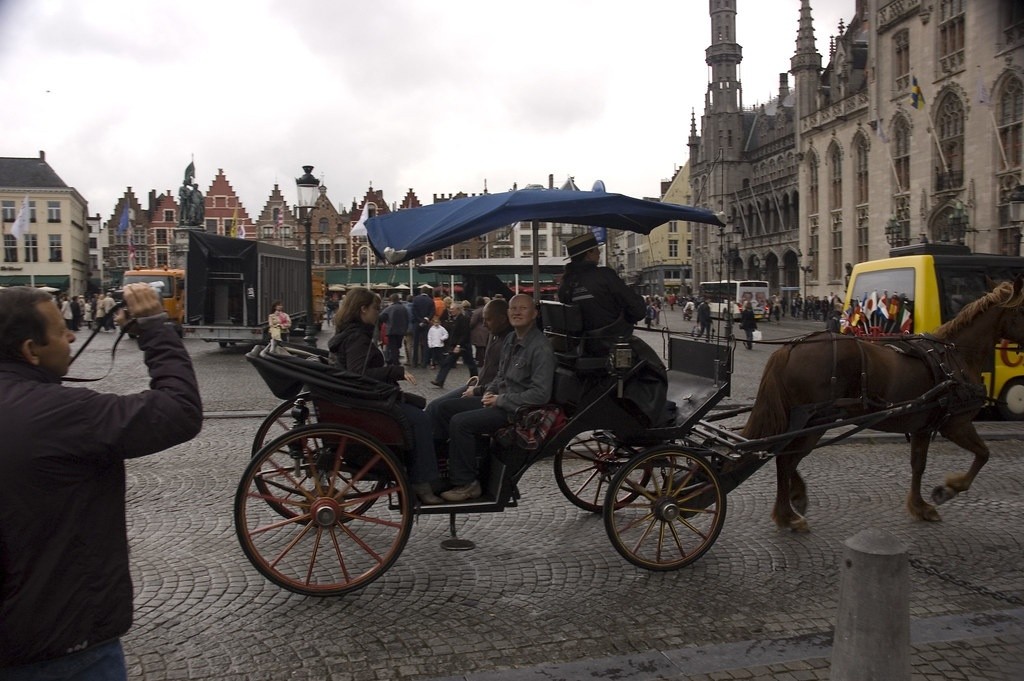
[
  {"xmin": 301, "ymin": 339, "xmax": 428, "ymax": 482},
  {"xmin": 477, "ymin": 368, "xmax": 579, "ymax": 467}
]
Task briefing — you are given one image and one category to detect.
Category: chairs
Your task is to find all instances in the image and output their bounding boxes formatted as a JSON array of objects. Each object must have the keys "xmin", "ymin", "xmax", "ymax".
[{"xmin": 538, "ymin": 297, "xmax": 631, "ymax": 399}]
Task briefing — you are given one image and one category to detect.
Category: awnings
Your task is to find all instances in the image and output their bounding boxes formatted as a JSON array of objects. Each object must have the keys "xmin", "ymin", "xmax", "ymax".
[{"xmin": 419, "ymin": 257, "xmax": 571, "ymax": 301}]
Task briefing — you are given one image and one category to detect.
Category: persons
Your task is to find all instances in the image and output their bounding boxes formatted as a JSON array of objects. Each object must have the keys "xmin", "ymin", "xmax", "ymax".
[
  {"xmin": 559, "ymin": 232, "xmax": 646, "ymax": 354},
  {"xmin": 328, "ymin": 288, "xmax": 445, "ymax": 504},
  {"xmin": 440, "ymin": 294, "xmax": 554, "ymax": 509},
  {"xmin": 645, "ymin": 294, "xmax": 842, "ymax": 350},
  {"xmin": 427, "ymin": 300, "xmax": 506, "ymax": 420},
  {"xmin": 269, "ymin": 301, "xmax": 292, "ymax": 341},
  {"xmin": 327, "ymin": 286, "xmax": 506, "ymax": 388},
  {"xmin": 0, "ymin": 282, "xmax": 204, "ymax": 681},
  {"xmin": 178, "ymin": 180, "xmax": 204, "ymax": 226},
  {"xmin": 457, "ymin": 345, "xmax": 460, "ymax": 348},
  {"xmin": 52, "ymin": 292, "xmax": 116, "ymax": 331}
]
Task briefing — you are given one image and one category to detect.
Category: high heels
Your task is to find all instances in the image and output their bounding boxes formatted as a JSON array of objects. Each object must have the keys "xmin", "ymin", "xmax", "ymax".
[{"xmin": 411, "ymin": 484, "xmax": 445, "ymax": 504}]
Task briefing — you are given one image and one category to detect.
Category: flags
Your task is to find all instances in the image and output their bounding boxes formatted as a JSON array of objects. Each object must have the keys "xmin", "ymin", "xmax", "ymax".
[
  {"xmin": 118, "ymin": 204, "xmax": 128, "ymax": 233},
  {"xmin": 185, "ymin": 162, "xmax": 194, "ymax": 184},
  {"xmin": 11, "ymin": 200, "xmax": 29, "ymax": 239},
  {"xmin": 840, "ymin": 290, "xmax": 913, "ymax": 334}
]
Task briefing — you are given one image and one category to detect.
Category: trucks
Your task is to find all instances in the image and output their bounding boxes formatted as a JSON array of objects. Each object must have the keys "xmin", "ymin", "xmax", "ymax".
[{"xmin": 182, "ymin": 229, "xmax": 309, "ymax": 349}]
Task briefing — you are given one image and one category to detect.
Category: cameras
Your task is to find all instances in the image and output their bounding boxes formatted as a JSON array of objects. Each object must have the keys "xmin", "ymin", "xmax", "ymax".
[{"xmin": 112, "ymin": 280, "xmax": 165, "ymax": 319}]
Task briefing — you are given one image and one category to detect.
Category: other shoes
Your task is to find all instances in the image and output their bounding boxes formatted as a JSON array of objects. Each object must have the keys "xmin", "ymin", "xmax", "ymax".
[
  {"xmin": 440, "ymin": 479, "xmax": 482, "ymax": 502},
  {"xmin": 430, "ymin": 381, "xmax": 443, "ymax": 386}
]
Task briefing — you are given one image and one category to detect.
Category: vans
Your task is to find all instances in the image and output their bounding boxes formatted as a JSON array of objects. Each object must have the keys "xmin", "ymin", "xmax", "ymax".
[{"xmin": 840, "ymin": 243, "xmax": 1024, "ymax": 421}]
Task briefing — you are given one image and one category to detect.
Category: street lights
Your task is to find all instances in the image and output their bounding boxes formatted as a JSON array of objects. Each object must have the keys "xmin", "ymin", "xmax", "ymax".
[
  {"xmin": 949, "ymin": 200, "xmax": 968, "ymax": 244},
  {"xmin": 798, "ymin": 247, "xmax": 813, "ymax": 319},
  {"xmin": 720, "ymin": 214, "xmax": 743, "ymax": 338},
  {"xmin": 293, "ymin": 166, "xmax": 320, "ymax": 349}
]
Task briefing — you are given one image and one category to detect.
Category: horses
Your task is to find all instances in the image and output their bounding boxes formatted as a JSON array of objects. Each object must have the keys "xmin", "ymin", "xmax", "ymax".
[{"xmin": 740, "ymin": 272, "xmax": 1024, "ymax": 533}]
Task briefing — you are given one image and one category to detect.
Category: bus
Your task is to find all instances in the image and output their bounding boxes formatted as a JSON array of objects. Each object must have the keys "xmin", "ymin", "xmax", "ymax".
[
  {"xmin": 697, "ymin": 279, "xmax": 769, "ymax": 321},
  {"xmin": 119, "ymin": 266, "xmax": 186, "ymax": 339}
]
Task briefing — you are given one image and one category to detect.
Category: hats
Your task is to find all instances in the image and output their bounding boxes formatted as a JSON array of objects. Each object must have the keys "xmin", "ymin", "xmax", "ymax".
[{"xmin": 562, "ymin": 231, "xmax": 605, "ymax": 260}]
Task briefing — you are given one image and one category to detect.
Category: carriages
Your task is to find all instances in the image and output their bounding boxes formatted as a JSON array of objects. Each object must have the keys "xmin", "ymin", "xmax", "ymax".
[{"xmin": 235, "ymin": 188, "xmax": 1024, "ymax": 597}]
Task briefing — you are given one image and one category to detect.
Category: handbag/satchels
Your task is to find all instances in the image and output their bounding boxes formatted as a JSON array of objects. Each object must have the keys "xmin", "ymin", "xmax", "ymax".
[
  {"xmin": 752, "ymin": 330, "xmax": 761, "ymax": 340},
  {"xmin": 691, "ymin": 326, "xmax": 701, "ymax": 339},
  {"xmin": 400, "ymin": 391, "xmax": 426, "ymax": 408}
]
